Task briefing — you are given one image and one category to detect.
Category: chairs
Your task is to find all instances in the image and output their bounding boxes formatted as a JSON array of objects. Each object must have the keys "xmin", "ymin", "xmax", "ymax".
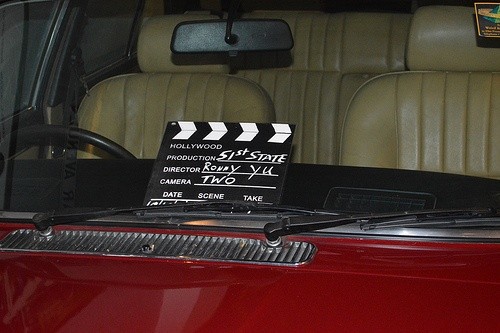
[
  {"xmin": 76, "ymin": 13, "xmax": 276, "ymax": 160},
  {"xmin": 338, "ymin": 5, "xmax": 500, "ymax": 180}
]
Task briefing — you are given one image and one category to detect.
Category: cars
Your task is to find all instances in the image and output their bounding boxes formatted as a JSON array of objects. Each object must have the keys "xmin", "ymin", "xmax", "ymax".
[{"xmin": 0, "ymin": 0, "xmax": 499, "ymax": 333}]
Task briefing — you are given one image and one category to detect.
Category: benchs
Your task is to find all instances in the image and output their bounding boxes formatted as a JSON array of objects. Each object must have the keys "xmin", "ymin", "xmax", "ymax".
[{"xmin": 222, "ymin": 11, "xmax": 416, "ymax": 167}]
